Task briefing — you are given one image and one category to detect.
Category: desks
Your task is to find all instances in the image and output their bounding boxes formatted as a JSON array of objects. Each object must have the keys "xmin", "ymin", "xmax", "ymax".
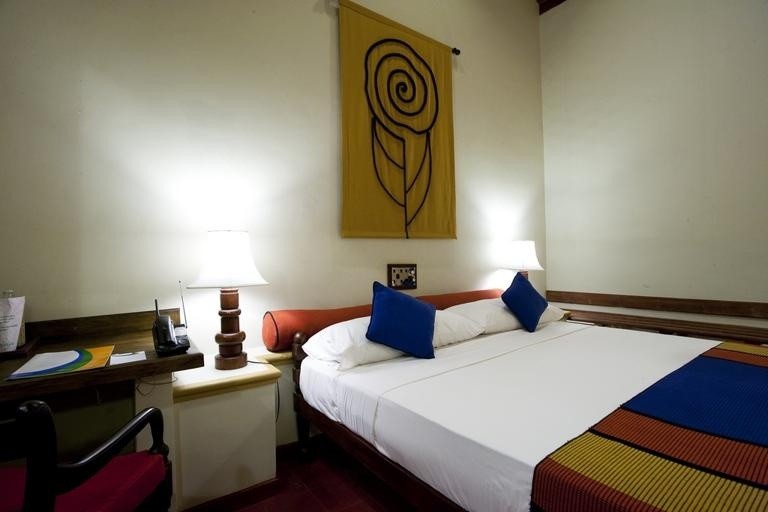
[{"xmin": 0, "ymin": 307, "xmax": 205, "ymax": 512}]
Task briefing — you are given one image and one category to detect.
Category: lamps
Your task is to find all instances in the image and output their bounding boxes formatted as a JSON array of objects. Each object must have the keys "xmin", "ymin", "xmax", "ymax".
[
  {"xmin": 498, "ymin": 240, "xmax": 544, "ymax": 280},
  {"xmin": 185, "ymin": 229, "xmax": 270, "ymax": 371}
]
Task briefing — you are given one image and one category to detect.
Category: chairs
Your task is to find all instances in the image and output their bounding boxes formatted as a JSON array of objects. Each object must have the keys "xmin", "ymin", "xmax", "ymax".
[{"xmin": 0, "ymin": 399, "xmax": 174, "ymax": 512}]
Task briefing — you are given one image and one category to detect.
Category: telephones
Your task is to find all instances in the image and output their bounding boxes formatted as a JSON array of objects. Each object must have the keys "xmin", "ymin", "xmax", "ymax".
[{"xmin": 152, "ymin": 299, "xmax": 190, "ymax": 357}]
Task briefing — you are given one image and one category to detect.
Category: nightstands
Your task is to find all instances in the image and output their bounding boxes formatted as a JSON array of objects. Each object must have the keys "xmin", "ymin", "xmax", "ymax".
[{"xmin": 173, "ymin": 350, "xmax": 297, "ymax": 512}]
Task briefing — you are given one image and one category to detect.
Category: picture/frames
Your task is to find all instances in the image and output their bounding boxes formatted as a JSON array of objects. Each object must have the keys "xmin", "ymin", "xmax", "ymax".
[{"xmin": 386, "ymin": 262, "xmax": 419, "ymax": 290}]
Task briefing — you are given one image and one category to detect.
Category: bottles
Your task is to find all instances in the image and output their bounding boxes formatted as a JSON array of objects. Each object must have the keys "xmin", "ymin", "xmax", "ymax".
[{"xmin": 0, "ymin": 289, "xmax": 26, "ymax": 348}]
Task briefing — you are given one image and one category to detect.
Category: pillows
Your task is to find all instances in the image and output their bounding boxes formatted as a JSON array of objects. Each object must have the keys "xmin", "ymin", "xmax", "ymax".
[
  {"xmin": 366, "ymin": 281, "xmax": 437, "ymax": 360},
  {"xmin": 300, "ymin": 309, "xmax": 486, "ymax": 373},
  {"xmin": 262, "ymin": 289, "xmax": 504, "ymax": 351},
  {"xmin": 500, "ymin": 271, "xmax": 549, "ymax": 332},
  {"xmin": 443, "ymin": 297, "xmax": 571, "ymax": 336}
]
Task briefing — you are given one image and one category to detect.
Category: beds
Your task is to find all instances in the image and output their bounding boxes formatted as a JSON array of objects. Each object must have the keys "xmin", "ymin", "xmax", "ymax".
[{"xmin": 291, "ymin": 290, "xmax": 768, "ymax": 512}]
